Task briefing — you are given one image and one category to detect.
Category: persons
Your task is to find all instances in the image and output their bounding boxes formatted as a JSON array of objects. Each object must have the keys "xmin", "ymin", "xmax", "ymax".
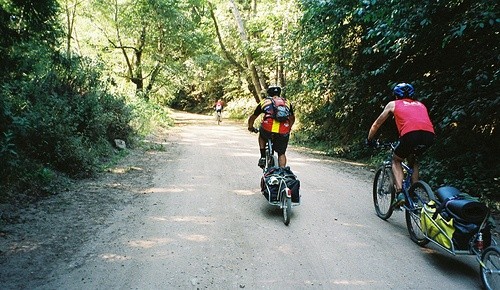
[
  {"xmin": 247, "ymin": 86, "xmax": 295, "ymax": 168},
  {"xmin": 213, "ymin": 99, "xmax": 224, "ymax": 122},
  {"xmin": 363, "ymin": 82, "xmax": 438, "ymax": 209}
]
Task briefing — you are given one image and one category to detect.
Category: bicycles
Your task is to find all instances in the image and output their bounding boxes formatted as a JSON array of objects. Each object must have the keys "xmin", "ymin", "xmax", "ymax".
[
  {"xmin": 366, "ymin": 138, "xmax": 500, "ymax": 290},
  {"xmin": 248, "ymin": 126, "xmax": 301, "ymax": 224},
  {"xmin": 215, "ymin": 112, "xmax": 222, "ymax": 125}
]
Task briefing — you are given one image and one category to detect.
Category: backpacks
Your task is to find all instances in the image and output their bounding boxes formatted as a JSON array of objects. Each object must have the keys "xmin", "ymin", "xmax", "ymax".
[{"xmin": 266, "ymin": 97, "xmax": 290, "ymax": 122}]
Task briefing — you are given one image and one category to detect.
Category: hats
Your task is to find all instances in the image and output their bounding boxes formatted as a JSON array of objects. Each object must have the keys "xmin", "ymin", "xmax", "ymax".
[{"xmin": 267, "ymin": 86, "xmax": 281, "ymax": 95}]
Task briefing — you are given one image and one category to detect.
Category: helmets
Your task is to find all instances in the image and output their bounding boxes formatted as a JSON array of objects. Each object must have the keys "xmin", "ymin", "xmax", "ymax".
[{"xmin": 393, "ymin": 82, "xmax": 415, "ymax": 97}]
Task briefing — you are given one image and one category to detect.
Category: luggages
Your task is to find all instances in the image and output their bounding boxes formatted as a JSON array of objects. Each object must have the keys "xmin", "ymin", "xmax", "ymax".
[
  {"xmin": 417, "ymin": 186, "xmax": 491, "ymax": 249},
  {"xmin": 260, "ymin": 167, "xmax": 300, "ymax": 203}
]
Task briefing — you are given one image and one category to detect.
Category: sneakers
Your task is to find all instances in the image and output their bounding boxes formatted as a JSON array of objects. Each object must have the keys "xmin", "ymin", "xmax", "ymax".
[
  {"xmin": 258, "ymin": 158, "xmax": 266, "ymax": 166},
  {"xmin": 412, "ymin": 198, "xmax": 419, "ymax": 207},
  {"xmin": 392, "ymin": 192, "xmax": 405, "ymax": 206}
]
